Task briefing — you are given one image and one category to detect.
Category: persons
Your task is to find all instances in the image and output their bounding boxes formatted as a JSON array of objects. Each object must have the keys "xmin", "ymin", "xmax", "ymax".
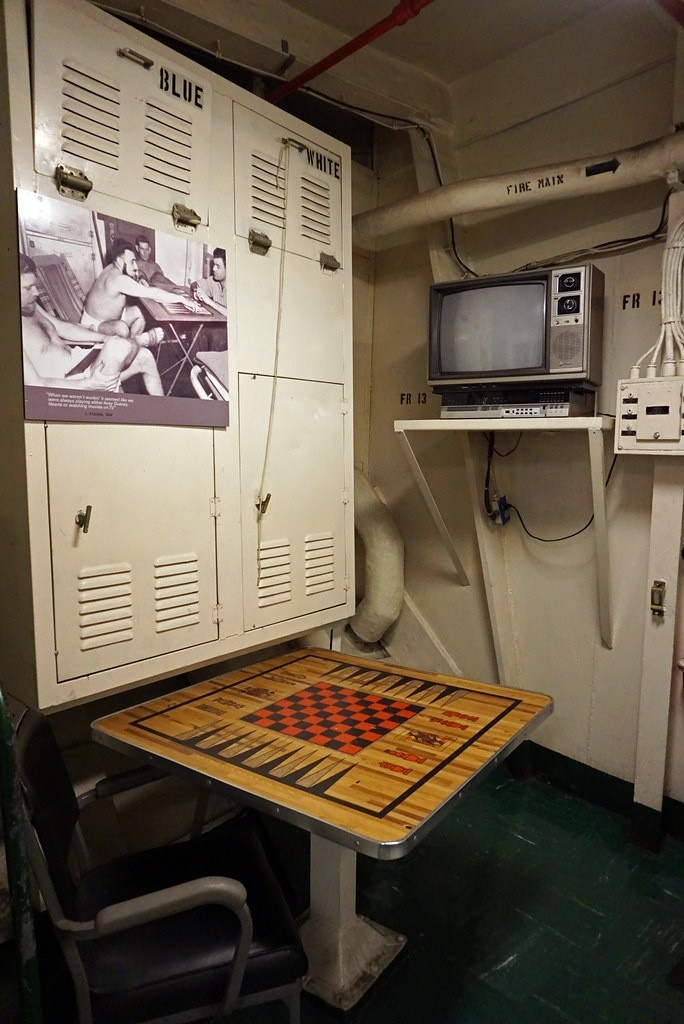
[{"xmin": 19, "ymin": 235, "xmax": 227, "ymax": 396}]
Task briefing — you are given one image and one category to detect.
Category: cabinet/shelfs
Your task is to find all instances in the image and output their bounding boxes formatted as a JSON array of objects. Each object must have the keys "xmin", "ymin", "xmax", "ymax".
[{"xmin": 0, "ymin": 0, "xmax": 355, "ymax": 715}]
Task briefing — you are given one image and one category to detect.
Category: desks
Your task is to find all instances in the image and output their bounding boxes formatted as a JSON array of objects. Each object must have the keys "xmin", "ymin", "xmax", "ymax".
[
  {"xmin": 91, "ymin": 645, "xmax": 555, "ymax": 1012},
  {"xmin": 139, "ymin": 285, "xmax": 227, "ymax": 397}
]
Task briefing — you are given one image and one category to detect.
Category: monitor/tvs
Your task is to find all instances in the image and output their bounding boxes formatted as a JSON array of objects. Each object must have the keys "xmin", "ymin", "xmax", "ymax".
[{"xmin": 427, "ymin": 262, "xmax": 604, "ymax": 395}]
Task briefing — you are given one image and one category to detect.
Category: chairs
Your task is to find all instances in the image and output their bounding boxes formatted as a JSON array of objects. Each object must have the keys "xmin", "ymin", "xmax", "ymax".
[
  {"xmin": 6, "ymin": 706, "xmax": 312, "ymax": 1024},
  {"xmin": 32, "ymin": 254, "xmax": 86, "ymax": 325}
]
